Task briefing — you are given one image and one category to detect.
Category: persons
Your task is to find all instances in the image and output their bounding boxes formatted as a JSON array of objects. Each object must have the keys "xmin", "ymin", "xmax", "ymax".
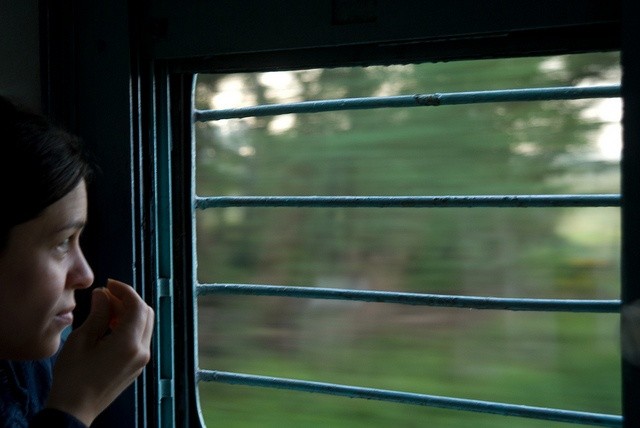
[{"xmin": 0, "ymin": 110, "xmax": 155, "ymax": 428}]
